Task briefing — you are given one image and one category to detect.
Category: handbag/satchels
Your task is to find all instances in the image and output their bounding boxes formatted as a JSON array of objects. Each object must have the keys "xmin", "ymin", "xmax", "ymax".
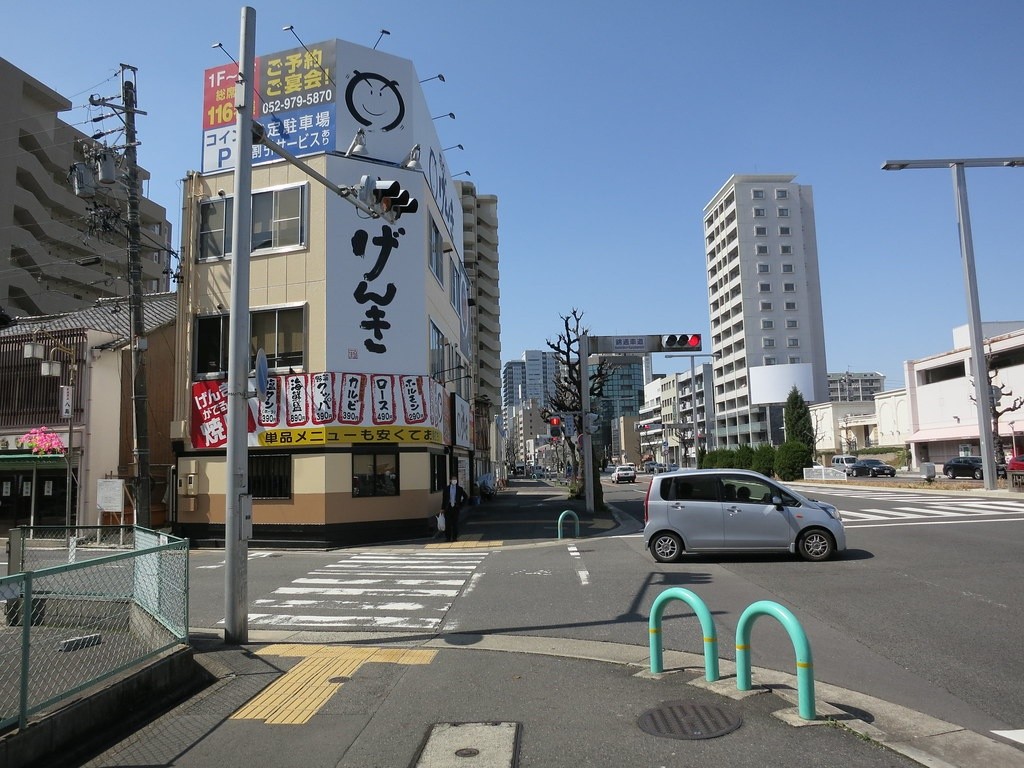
[{"xmin": 434, "ymin": 512, "xmax": 446, "ymax": 533}]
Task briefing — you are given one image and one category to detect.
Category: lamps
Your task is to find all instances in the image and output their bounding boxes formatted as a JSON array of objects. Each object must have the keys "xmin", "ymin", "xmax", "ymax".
[
  {"xmin": 419, "ymin": 74, "xmax": 445, "ymax": 83},
  {"xmin": 24, "ymin": 328, "xmax": 73, "ymax": 376},
  {"xmin": 373, "ymin": 29, "xmax": 390, "ymax": 49},
  {"xmin": 282, "ymin": 25, "xmax": 304, "ymax": 46},
  {"xmin": 344, "ymin": 127, "xmax": 369, "ymax": 158},
  {"xmin": 211, "ymin": 43, "xmax": 235, "ymax": 62},
  {"xmin": 432, "ymin": 113, "xmax": 455, "ymax": 120},
  {"xmin": 434, "ymin": 365, "xmax": 491, "ymax": 408},
  {"xmin": 443, "ymin": 144, "xmax": 464, "ymax": 152},
  {"xmin": 400, "ymin": 143, "xmax": 422, "ymax": 169},
  {"xmin": 451, "ymin": 171, "xmax": 471, "ymax": 177}
]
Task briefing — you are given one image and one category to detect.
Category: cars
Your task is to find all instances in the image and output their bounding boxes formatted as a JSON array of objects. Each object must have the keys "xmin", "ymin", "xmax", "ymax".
[
  {"xmin": 609, "ymin": 466, "xmax": 637, "ymax": 483},
  {"xmin": 942, "ymin": 457, "xmax": 1006, "ymax": 480},
  {"xmin": 851, "ymin": 459, "xmax": 896, "ymax": 477},
  {"xmin": 1008, "ymin": 454, "xmax": 1024, "ymax": 484},
  {"xmin": 811, "ymin": 461, "xmax": 823, "ymax": 469},
  {"xmin": 517, "ymin": 465, "xmax": 557, "ymax": 476},
  {"xmin": 644, "ymin": 458, "xmax": 679, "ymax": 473}
]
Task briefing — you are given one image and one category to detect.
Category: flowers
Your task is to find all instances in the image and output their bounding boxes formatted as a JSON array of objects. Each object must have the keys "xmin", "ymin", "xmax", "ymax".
[{"xmin": 19, "ymin": 427, "xmax": 66, "ymax": 454}]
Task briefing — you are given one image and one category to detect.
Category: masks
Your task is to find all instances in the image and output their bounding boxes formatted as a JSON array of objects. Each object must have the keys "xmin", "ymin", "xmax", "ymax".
[{"xmin": 450, "ymin": 480, "xmax": 458, "ymax": 486}]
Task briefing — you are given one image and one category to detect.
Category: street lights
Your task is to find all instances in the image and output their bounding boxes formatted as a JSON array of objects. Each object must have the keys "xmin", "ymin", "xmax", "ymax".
[{"xmin": 666, "ymin": 353, "xmax": 722, "ymax": 468}]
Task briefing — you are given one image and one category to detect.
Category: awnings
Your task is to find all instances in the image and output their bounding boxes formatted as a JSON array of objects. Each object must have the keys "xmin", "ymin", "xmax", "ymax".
[{"xmin": 0, "ymin": 453, "xmax": 64, "ymax": 461}]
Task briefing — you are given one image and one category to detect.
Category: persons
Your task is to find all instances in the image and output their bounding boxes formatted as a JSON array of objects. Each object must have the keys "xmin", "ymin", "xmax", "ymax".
[
  {"xmin": 737, "ymin": 486, "xmax": 751, "ymax": 499},
  {"xmin": 724, "ymin": 483, "xmax": 736, "ymax": 502},
  {"xmin": 441, "ymin": 476, "xmax": 468, "ymax": 541}
]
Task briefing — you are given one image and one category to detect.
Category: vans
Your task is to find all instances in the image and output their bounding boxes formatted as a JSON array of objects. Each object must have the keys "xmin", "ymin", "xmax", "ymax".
[
  {"xmin": 643, "ymin": 467, "xmax": 846, "ymax": 563},
  {"xmin": 831, "ymin": 455, "xmax": 860, "ymax": 475},
  {"xmin": 626, "ymin": 462, "xmax": 636, "ymax": 471}
]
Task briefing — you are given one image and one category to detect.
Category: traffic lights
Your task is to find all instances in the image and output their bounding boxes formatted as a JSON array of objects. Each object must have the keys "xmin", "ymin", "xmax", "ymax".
[
  {"xmin": 662, "ymin": 332, "xmax": 704, "ymax": 351},
  {"xmin": 548, "ymin": 415, "xmax": 562, "ymax": 439},
  {"xmin": 636, "ymin": 423, "xmax": 650, "ymax": 431},
  {"xmin": 373, "ymin": 180, "xmax": 421, "ymax": 216},
  {"xmin": 588, "ymin": 412, "xmax": 600, "ymax": 434}
]
